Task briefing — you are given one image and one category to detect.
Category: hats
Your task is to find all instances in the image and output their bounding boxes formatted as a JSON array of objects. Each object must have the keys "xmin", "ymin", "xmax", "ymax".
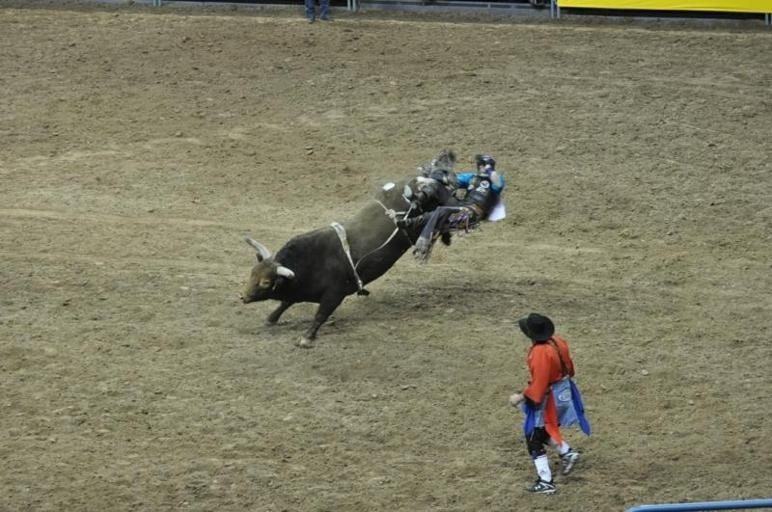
[{"xmin": 518, "ymin": 312, "xmax": 555, "ymax": 342}]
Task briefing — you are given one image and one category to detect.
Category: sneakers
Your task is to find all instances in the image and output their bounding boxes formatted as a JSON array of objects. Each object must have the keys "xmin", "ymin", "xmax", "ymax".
[
  {"xmin": 558, "ymin": 448, "xmax": 579, "ymax": 475},
  {"xmin": 522, "ymin": 476, "xmax": 557, "ymax": 494}
]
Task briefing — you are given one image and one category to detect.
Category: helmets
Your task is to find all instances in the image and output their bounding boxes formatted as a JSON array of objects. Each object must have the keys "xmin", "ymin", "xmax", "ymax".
[{"xmin": 475, "ymin": 154, "xmax": 496, "ymax": 171}]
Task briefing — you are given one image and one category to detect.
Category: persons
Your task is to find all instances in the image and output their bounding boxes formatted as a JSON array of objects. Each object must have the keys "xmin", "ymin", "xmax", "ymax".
[
  {"xmin": 508, "ymin": 313, "xmax": 589, "ymax": 496},
  {"xmin": 304, "ymin": 1, "xmax": 332, "ymax": 22},
  {"xmin": 394, "ymin": 154, "xmax": 504, "ymax": 261}
]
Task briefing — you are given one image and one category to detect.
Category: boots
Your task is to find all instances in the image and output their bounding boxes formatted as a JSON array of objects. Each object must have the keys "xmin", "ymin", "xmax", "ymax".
[
  {"xmin": 396, "ymin": 214, "xmax": 429, "ymax": 233},
  {"xmin": 403, "ymin": 184, "xmax": 431, "ymax": 210}
]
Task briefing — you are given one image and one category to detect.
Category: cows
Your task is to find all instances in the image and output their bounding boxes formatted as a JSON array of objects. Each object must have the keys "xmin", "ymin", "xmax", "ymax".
[{"xmin": 235, "ymin": 146, "xmax": 456, "ymax": 346}]
